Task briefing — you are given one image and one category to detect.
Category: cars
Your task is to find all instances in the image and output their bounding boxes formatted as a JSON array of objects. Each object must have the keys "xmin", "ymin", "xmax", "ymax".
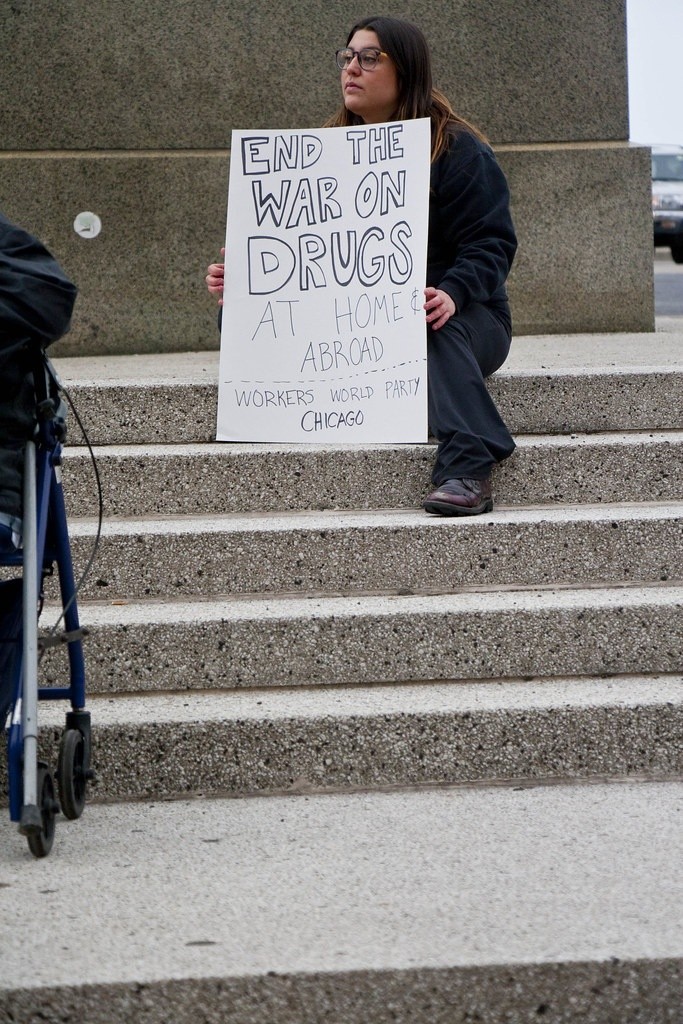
[{"xmin": 650, "ymin": 142, "xmax": 683, "ymax": 264}]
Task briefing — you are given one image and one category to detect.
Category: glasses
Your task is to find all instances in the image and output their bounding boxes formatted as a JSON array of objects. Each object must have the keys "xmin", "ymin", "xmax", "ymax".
[{"xmin": 335, "ymin": 48, "xmax": 389, "ymax": 71}]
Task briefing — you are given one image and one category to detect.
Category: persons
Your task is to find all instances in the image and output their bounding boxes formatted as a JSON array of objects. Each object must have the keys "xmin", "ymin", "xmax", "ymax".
[{"xmin": 207, "ymin": 15, "xmax": 518, "ymax": 514}]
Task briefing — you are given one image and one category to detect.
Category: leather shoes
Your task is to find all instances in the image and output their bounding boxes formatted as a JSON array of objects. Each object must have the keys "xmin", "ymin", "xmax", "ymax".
[{"xmin": 424, "ymin": 477, "xmax": 493, "ymax": 514}]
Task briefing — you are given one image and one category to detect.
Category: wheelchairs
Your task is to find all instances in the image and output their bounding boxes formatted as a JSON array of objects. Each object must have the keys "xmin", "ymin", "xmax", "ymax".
[{"xmin": 0, "ymin": 349, "xmax": 106, "ymax": 859}]
